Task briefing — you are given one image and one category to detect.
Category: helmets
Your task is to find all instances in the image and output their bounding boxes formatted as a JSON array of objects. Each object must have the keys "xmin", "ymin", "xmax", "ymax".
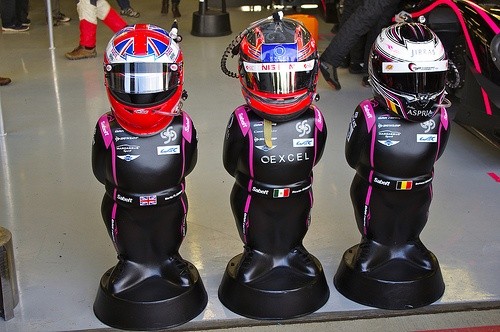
[
  {"xmin": 238, "ymin": 18, "xmax": 319, "ymax": 123},
  {"xmin": 104, "ymin": 23, "xmax": 185, "ymax": 137},
  {"xmin": 368, "ymin": 21, "xmax": 450, "ymax": 121}
]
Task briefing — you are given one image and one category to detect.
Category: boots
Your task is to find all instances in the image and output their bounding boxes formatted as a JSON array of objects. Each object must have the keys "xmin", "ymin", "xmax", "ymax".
[
  {"xmin": 161, "ymin": 0, "xmax": 169, "ymax": 14},
  {"xmin": 171, "ymin": 0, "xmax": 181, "ymax": 18}
]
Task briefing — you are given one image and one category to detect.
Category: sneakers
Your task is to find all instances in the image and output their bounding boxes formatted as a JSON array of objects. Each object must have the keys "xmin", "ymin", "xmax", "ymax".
[{"xmin": 65, "ymin": 45, "xmax": 97, "ymax": 59}]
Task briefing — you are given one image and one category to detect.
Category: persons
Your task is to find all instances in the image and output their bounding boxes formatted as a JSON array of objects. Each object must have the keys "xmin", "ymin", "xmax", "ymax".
[
  {"xmin": 317, "ymin": 0, "xmax": 397, "ymax": 90},
  {"xmin": 345, "ymin": 23, "xmax": 449, "ymax": 271},
  {"xmin": 321, "ymin": 0, "xmax": 366, "ymax": 74},
  {"xmin": 118, "ymin": 0, "xmax": 140, "ymax": 18},
  {"xmin": 44, "ymin": 0, "xmax": 71, "ymax": 27},
  {"xmin": 161, "ymin": 0, "xmax": 181, "ymax": 17},
  {"xmin": 0, "ymin": 0, "xmax": 31, "ymax": 32},
  {"xmin": 0, "ymin": 77, "xmax": 12, "ymax": 86},
  {"xmin": 65, "ymin": 0, "xmax": 131, "ymax": 60},
  {"xmin": 91, "ymin": 23, "xmax": 195, "ymax": 294},
  {"xmin": 221, "ymin": 16, "xmax": 320, "ymax": 282}
]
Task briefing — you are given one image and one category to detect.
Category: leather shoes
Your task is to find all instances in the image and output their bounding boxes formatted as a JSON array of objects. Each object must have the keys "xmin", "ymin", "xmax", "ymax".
[
  {"xmin": 320, "ymin": 61, "xmax": 340, "ymax": 91},
  {"xmin": 349, "ymin": 62, "xmax": 367, "ymax": 74}
]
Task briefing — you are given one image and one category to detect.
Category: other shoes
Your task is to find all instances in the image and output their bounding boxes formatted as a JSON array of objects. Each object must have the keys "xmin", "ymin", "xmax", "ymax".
[
  {"xmin": 0, "ymin": 77, "xmax": 11, "ymax": 86},
  {"xmin": 54, "ymin": 13, "xmax": 70, "ymax": 21},
  {"xmin": 45, "ymin": 18, "xmax": 61, "ymax": 25},
  {"xmin": 1, "ymin": 22, "xmax": 30, "ymax": 32},
  {"xmin": 120, "ymin": 6, "xmax": 140, "ymax": 18},
  {"xmin": 20, "ymin": 19, "xmax": 32, "ymax": 25}
]
